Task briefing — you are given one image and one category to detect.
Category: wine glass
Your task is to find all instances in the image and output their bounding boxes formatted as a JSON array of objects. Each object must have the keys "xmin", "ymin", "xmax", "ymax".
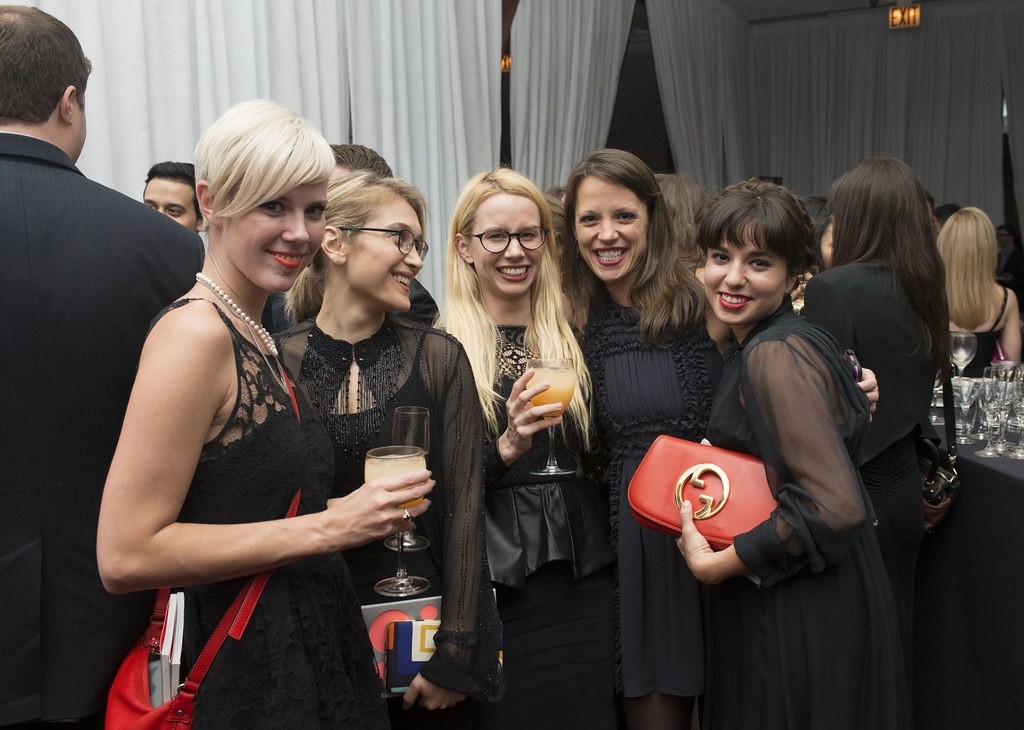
[
  {"xmin": 930, "ymin": 332, "xmax": 1024, "ymax": 461},
  {"xmin": 385, "ymin": 406, "xmax": 430, "ymax": 551},
  {"xmin": 365, "ymin": 445, "xmax": 430, "ymax": 597},
  {"xmin": 525, "ymin": 358, "xmax": 578, "ymax": 476}
]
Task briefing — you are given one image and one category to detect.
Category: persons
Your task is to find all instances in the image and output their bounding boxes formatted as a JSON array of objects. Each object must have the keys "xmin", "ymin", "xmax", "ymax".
[
  {"xmin": 269, "ymin": 171, "xmax": 502, "ymax": 730},
  {"xmin": 0, "ymin": 6, "xmax": 204, "ymax": 730},
  {"xmin": 96, "ymin": 95, "xmax": 436, "ymax": 730},
  {"xmin": 804, "ymin": 155, "xmax": 1024, "ymax": 730},
  {"xmin": 143, "ymin": 160, "xmax": 205, "ymax": 234},
  {"xmin": 431, "ymin": 148, "xmax": 912, "ymax": 730},
  {"xmin": 271, "ymin": 143, "xmax": 440, "ymax": 325}
]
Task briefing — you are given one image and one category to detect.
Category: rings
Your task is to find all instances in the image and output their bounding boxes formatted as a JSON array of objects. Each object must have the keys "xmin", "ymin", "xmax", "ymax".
[{"xmin": 402, "ymin": 508, "xmax": 411, "ymax": 522}]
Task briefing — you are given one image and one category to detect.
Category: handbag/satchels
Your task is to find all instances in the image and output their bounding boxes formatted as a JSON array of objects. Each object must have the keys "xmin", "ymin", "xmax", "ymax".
[
  {"xmin": 917, "ymin": 457, "xmax": 960, "ymax": 531},
  {"xmin": 104, "ymin": 620, "xmax": 195, "ymax": 730},
  {"xmin": 628, "ymin": 436, "xmax": 778, "ymax": 549}
]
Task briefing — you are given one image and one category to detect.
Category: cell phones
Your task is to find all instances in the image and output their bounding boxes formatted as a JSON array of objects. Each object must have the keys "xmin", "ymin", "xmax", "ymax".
[{"xmin": 844, "ymin": 349, "xmax": 862, "ymax": 384}]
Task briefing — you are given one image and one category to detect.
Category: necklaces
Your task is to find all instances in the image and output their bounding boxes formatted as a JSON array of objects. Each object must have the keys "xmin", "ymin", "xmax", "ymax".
[{"xmin": 196, "ymin": 273, "xmax": 293, "ymax": 396}]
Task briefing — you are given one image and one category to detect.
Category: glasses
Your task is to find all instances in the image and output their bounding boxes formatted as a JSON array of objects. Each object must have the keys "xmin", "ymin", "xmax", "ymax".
[
  {"xmin": 466, "ymin": 225, "xmax": 550, "ymax": 253},
  {"xmin": 995, "ymin": 233, "xmax": 1012, "ymax": 239},
  {"xmin": 338, "ymin": 226, "xmax": 428, "ymax": 262}
]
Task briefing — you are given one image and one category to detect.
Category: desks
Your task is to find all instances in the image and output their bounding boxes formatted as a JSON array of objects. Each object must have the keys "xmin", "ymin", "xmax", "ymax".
[{"xmin": 918, "ymin": 413, "xmax": 1024, "ymax": 730}]
